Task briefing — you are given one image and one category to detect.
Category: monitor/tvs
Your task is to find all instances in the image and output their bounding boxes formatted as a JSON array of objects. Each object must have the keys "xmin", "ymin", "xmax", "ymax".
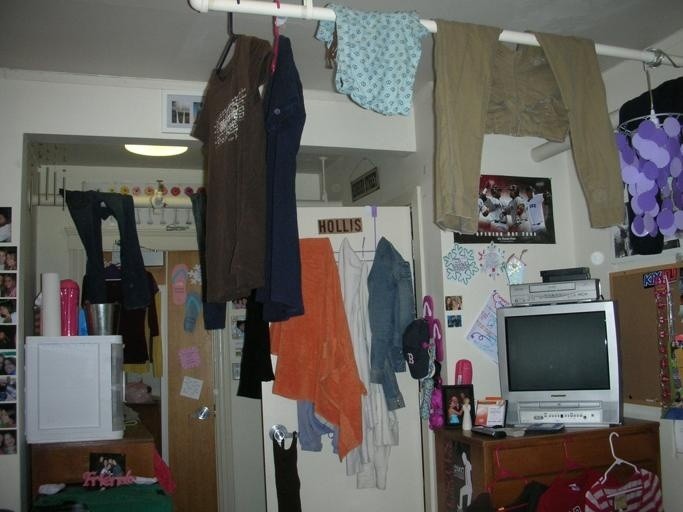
[{"xmin": 496, "ymin": 301, "xmax": 623, "ymax": 428}]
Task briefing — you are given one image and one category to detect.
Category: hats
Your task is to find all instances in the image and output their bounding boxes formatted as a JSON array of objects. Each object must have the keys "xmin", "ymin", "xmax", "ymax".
[{"xmin": 402, "ymin": 319, "xmax": 430, "ymax": 380}]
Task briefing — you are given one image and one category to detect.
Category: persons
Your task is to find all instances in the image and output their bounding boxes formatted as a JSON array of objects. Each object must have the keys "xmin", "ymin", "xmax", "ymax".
[
  {"xmin": 477, "ymin": 198, "xmax": 489, "ymax": 219},
  {"xmin": 1, "ymin": 251, "xmax": 15, "ymax": 271},
  {"xmin": 2, "ymin": 434, "xmax": 16, "ymax": 454},
  {"xmin": 525, "ymin": 186, "xmax": 551, "ymax": 232},
  {"xmin": 96, "ymin": 457, "xmax": 124, "ymax": 477},
  {"xmin": 0, "ymin": 352, "xmax": 16, "ymax": 373},
  {"xmin": 1, "ymin": 407, "xmax": 15, "ymax": 427},
  {"xmin": 0, "ymin": 275, "xmax": 15, "ymax": 298},
  {"xmin": 480, "ymin": 183, "xmax": 512, "ymax": 233},
  {"xmin": 463, "ymin": 399, "xmax": 472, "ymax": 430},
  {"xmin": 0, "ymin": 302, "xmax": 15, "ymax": 324},
  {"xmin": 506, "ymin": 185, "xmax": 531, "ymax": 232},
  {"xmin": 448, "ymin": 397, "xmax": 461, "ymax": 425}
]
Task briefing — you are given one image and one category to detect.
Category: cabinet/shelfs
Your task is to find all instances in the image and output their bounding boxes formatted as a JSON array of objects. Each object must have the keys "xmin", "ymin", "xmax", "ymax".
[
  {"xmin": 434, "ymin": 422, "xmax": 663, "ymax": 512},
  {"xmin": 32, "ymin": 441, "xmax": 155, "ymax": 495},
  {"xmin": 125, "ymin": 401, "xmax": 160, "ymax": 434},
  {"xmin": 23, "ymin": 336, "xmax": 124, "ymax": 444},
  {"xmin": 212, "ymin": 207, "xmax": 432, "ymax": 510}
]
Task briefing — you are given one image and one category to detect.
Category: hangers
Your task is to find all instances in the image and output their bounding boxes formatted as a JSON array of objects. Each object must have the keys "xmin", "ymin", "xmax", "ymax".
[
  {"xmin": 560, "ymin": 437, "xmax": 594, "ymax": 497},
  {"xmin": 272, "ymin": 0, "xmax": 281, "ymax": 73},
  {"xmin": 212, "ymin": 1, "xmax": 242, "ymax": 74},
  {"xmin": 488, "ymin": 442, "xmax": 531, "ymax": 512},
  {"xmin": 332, "ymin": 237, "xmax": 376, "ymax": 263},
  {"xmin": 590, "ymin": 432, "xmax": 646, "ymax": 499},
  {"xmin": 617, "ymin": 46, "xmax": 683, "ymax": 135}
]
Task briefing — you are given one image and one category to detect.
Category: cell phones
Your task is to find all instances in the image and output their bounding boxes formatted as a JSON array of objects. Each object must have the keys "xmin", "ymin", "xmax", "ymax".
[{"xmin": 526, "ymin": 423, "xmax": 564, "ymax": 434}]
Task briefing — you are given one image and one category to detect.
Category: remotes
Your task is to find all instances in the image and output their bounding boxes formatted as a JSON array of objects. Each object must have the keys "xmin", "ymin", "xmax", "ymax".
[{"xmin": 471, "ymin": 426, "xmax": 497, "ymax": 437}]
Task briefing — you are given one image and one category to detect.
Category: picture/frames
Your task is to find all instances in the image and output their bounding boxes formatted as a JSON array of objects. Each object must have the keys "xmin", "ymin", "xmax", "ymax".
[
  {"xmin": 454, "ymin": 174, "xmax": 556, "ymax": 244},
  {"xmin": 231, "ymin": 297, "xmax": 247, "ymax": 310},
  {"xmin": 442, "ymin": 385, "xmax": 475, "ymax": 428},
  {"xmin": 162, "ymin": 89, "xmax": 203, "ymax": 134},
  {"xmin": 230, "ymin": 315, "xmax": 246, "ymax": 339},
  {"xmin": 87, "ymin": 448, "xmax": 124, "ymax": 478},
  {"xmin": 232, "ymin": 363, "xmax": 240, "ymax": 379},
  {"xmin": 229, "ymin": 340, "xmax": 244, "ymax": 362}
]
